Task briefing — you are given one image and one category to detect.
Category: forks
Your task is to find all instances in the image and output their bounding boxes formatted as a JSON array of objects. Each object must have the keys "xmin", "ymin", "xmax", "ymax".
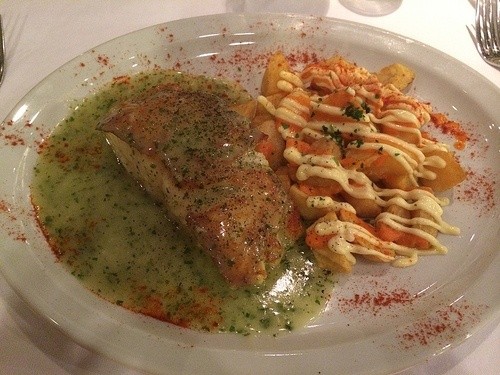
[{"xmin": 474, "ymin": 1, "xmax": 500, "ymax": 67}]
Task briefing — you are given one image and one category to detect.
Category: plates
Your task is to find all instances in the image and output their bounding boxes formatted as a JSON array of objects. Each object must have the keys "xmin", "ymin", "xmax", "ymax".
[{"xmin": 0, "ymin": 14, "xmax": 500, "ymax": 374}]
